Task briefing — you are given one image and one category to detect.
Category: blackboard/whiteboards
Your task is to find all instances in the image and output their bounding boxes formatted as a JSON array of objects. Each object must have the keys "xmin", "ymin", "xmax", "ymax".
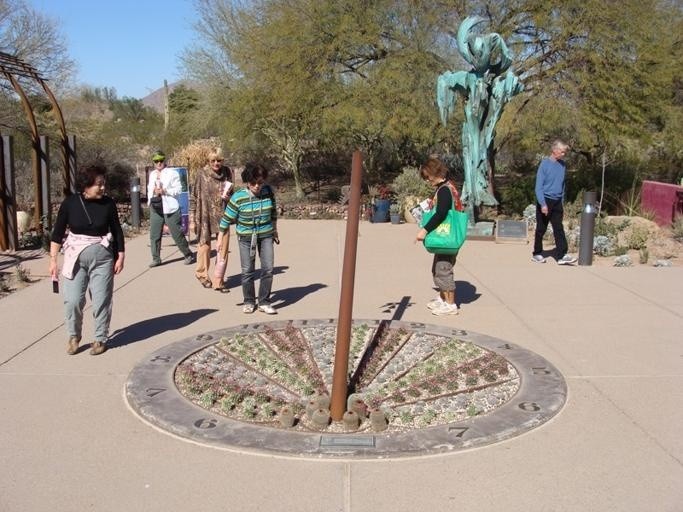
[{"xmin": 497, "ymin": 220, "xmax": 528, "ymax": 238}]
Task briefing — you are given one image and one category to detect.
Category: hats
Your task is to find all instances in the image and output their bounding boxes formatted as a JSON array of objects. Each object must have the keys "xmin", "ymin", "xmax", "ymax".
[{"xmin": 152, "ymin": 150, "xmax": 165, "ymax": 161}]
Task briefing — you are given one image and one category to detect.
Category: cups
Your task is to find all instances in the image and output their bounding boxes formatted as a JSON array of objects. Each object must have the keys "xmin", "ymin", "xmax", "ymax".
[{"xmin": 223, "ymin": 180, "xmax": 234, "ymax": 199}]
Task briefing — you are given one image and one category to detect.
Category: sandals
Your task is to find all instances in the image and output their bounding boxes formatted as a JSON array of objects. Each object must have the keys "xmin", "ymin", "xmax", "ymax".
[
  {"xmin": 195, "ymin": 272, "xmax": 212, "ymax": 288},
  {"xmin": 213, "ymin": 285, "xmax": 230, "ymax": 293}
]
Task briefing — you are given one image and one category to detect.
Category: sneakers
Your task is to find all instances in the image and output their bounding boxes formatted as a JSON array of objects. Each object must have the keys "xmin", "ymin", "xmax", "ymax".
[
  {"xmin": 427, "ymin": 299, "xmax": 458, "ymax": 316},
  {"xmin": 558, "ymin": 254, "xmax": 578, "ymax": 265},
  {"xmin": 149, "ymin": 261, "xmax": 161, "ymax": 267},
  {"xmin": 66, "ymin": 336, "xmax": 82, "ymax": 354},
  {"xmin": 185, "ymin": 256, "xmax": 196, "ymax": 264},
  {"xmin": 90, "ymin": 340, "xmax": 105, "ymax": 355},
  {"xmin": 243, "ymin": 304, "xmax": 255, "ymax": 313},
  {"xmin": 531, "ymin": 254, "xmax": 546, "ymax": 263},
  {"xmin": 258, "ymin": 305, "xmax": 278, "ymax": 314}
]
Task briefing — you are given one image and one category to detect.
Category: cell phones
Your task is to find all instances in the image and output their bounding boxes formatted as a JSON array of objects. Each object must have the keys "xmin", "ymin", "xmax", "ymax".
[{"xmin": 52, "ymin": 281, "xmax": 59, "ymax": 293}]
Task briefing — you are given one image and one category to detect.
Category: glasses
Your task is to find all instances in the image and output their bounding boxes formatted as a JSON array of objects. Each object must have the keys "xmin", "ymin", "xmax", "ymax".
[
  {"xmin": 250, "ymin": 180, "xmax": 265, "ymax": 187},
  {"xmin": 154, "ymin": 160, "xmax": 163, "ymax": 163}
]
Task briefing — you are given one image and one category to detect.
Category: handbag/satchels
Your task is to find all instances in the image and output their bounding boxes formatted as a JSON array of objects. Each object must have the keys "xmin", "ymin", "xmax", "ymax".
[
  {"xmin": 151, "ymin": 196, "xmax": 162, "ymax": 209},
  {"xmin": 420, "ymin": 207, "xmax": 468, "ymax": 256}
]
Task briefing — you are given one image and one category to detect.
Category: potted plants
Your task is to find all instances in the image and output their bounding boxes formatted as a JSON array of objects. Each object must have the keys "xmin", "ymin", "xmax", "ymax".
[
  {"xmin": 17, "ymin": 203, "xmax": 35, "ymax": 235},
  {"xmin": 388, "ymin": 204, "xmax": 402, "ymax": 224},
  {"xmin": 368, "ymin": 186, "xmax": 390, "ymax": 222},
  {"xmin": 390, "ymin": 164, "xmax": 437, "ymax": 223}
]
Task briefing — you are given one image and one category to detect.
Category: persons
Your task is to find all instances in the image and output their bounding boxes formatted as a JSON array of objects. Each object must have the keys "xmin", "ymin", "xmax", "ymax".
[
  {"xmin": 531, "ymin": 138, "xmax": 578, "ymax": 265},
  {"xmin": 215, "ymin": 162, "xmax": 280, "ymax": 315},
  {"xmin": 188, "ymin": 148, "xmax": 234, "ymax": 294},
  {"xmin": 147, "ymin": 150, "xmax": 195, "ymax": 268},
  {"xmin": 49, "ymin": 167, "xmax": 125, "ymax": 355},
  {"xmin": 416, "ymin": 159, "xmax": 463, "ymax": 316}
]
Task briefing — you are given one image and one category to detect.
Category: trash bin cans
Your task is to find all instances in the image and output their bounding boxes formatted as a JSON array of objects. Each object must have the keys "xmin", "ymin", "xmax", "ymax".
[{"xmin": 371, "ymin": 200, "xmax": 389, "ymax": 223}]
